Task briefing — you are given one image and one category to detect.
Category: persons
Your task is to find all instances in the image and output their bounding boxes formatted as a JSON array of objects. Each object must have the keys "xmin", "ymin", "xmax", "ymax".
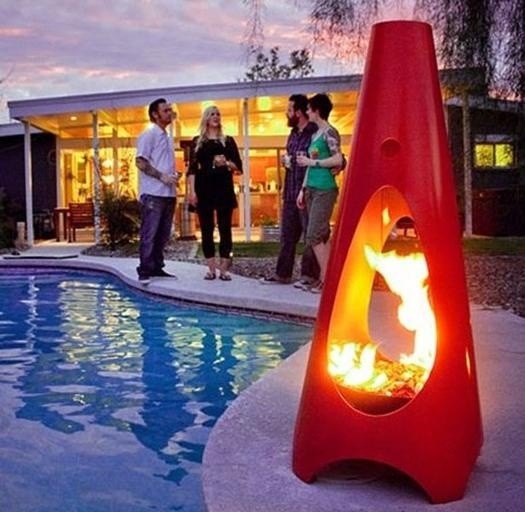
[
  {"xmin": 297, "ymin": 91, "xmax": 343, "ymax": 292},
  {"xmin": 259, "ymin": 94, "xmax": 346, "ymax": 295},
  {"xmin": 186, "ymin": 104, "xmax": 243, "ymax": 282},
  {"xmin": 136, "ymin": 99, "xmax": 178, "ymax": 285}
]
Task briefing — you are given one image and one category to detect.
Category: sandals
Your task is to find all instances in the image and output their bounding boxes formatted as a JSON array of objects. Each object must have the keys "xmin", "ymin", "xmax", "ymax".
[
  {"xmin": 203, "ymin": 272, "xmax": 217, "ymax": 280},
  {"xmin": 218, "ymin": 272, "xmax": 232, "ymax": 282}
]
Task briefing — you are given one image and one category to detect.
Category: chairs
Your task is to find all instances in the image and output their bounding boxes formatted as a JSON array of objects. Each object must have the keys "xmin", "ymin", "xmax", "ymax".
[{"xmin": 70, "ymin": 204, "xmax": 96, "ymax": 242}]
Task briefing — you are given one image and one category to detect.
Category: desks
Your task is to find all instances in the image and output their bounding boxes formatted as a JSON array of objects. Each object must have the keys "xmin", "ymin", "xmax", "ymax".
[{"xmin": 54, "ymin": 206, "xmax": 76, "ymax": 241}]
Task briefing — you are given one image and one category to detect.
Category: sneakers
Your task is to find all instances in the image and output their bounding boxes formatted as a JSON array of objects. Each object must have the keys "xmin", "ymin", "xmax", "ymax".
[
  {"xmin": 138, "ymin": 271, "xmax": 151, "ymax": 284},
  {"xmin": 151, "ymin": 269, "xmax": 177, "ymax": 280},
  {"xmin": 258, "ymin": 273, "xmax": 293, "ymax": 285},
  {"xmin": 301, "ymin": 281, "xmax": 321, "ymax": 292},
  {"xmin": 310, "ymin": 283, "xmax": 324, "ymax": 293},
  {"xmin": 293, "ymin": 279, "xmax": 316, "ymax": 288}
]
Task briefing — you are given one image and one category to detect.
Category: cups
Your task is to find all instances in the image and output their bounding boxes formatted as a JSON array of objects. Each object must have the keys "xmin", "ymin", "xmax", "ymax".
[{"xmin": 296, "ymin": 151, "xmax": 306, "ymax": 157}]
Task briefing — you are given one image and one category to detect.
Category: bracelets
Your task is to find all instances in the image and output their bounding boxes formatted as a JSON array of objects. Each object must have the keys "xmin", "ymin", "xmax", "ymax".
[{"xmin": 315, "ymin": 159, "xmax": 319, "ymax": 169}]
